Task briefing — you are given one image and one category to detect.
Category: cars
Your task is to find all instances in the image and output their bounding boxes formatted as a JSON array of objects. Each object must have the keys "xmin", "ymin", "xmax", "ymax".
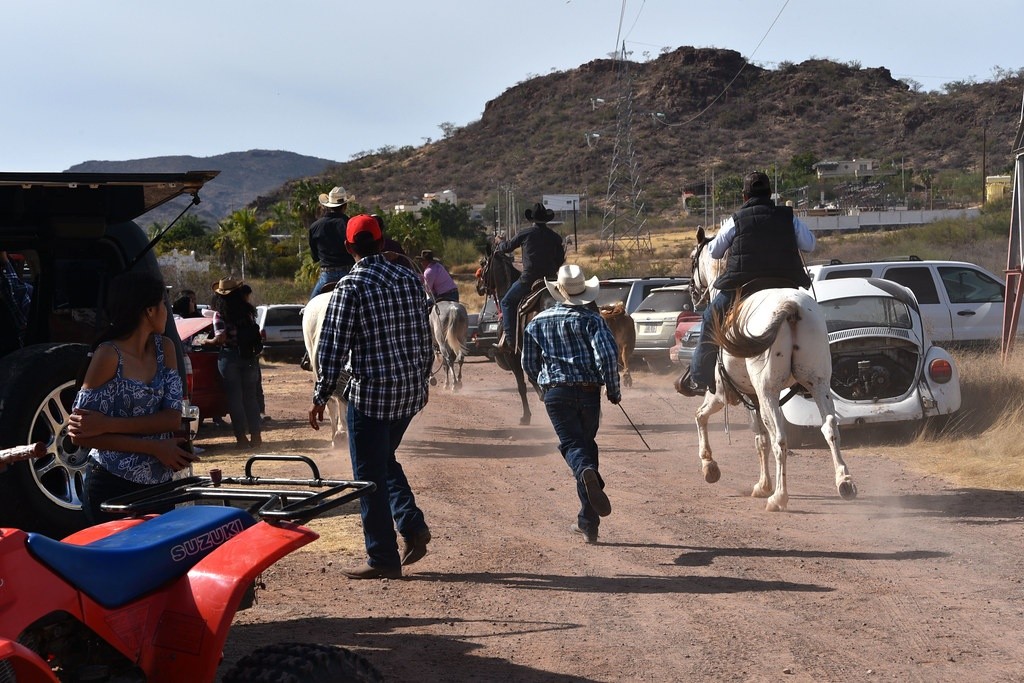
[
  {"xmin": 174, "ymin": 315, "xmax": 230, "ymax": 426},
  {"xmin": 465, "ymin": 313, "xmax": 488, "ymax": 357},
  {"xmin": 668, "ymin": 309, "xmax": 703, "ymax": 369},
  {"xmin": 629, "ymin": 285, "xmax": 697, "ymax": 375},
  {"xmin": 746, "ymin": 277, "xmax": 962, "ymax": 448}
]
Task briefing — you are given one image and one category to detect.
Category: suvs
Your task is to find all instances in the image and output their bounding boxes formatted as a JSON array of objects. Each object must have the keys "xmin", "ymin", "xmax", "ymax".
[
  {"xmin": 473, "ymin": 295, "xmax": 512, "ymax": 371},
  {"xmin": 0, "ymin": 168, "xmax": 224, "ymax": 540},
  {"xmin": 800, "ymin": 254, "xmax": 1024, "ymax": 350},
  {"xmin": 253, "ymin": 302, "xmax": 307, "ymax": 361},
  {"xmin": 596, "ymin": 274, "xmax": 692, "ymax": 316}
]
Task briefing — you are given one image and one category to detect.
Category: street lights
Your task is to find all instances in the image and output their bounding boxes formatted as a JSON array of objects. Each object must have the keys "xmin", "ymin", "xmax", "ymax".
[{"xmin": 240, "ymin": 248, "xmax": 258, "ymax": 280}]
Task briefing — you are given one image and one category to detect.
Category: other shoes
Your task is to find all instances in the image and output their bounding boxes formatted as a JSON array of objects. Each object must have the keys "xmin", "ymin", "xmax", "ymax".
[
  {"xmin": 251, "ymin": 432, "xmax": 262, "ymax": 447},
  {"xmin": 401, "ymin": 530, "xmax": 431, "ymax": 565},
  {"xmin": 339, "ymin": 560, "xmax": 401, "ymax": 579},
  {"xmin": 583, "ymin": 469, "xmax": 612, "ymax": 516},
  {"xmin": 686, "ymin": 377, "xmax": 707, "ymax": 396},
  {"xmin": 237, "ymin": 435, "xmax": 251, "ymax": 447},
  {"xmin": 571, "ymin": 522, "xmax": 598, "ymax": 543}
]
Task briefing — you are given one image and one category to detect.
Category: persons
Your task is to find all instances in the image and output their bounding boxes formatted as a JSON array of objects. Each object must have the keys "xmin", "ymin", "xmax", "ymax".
[
  {"xmin": 175, "ymin": 276, "xmax": 265, "ymax": 449},
  {"xmin": 307, "ymin": 187, "xmax": 386, "ymax": 300},
  {"xmin": 370, "ymin": 214, "xmax": 410, "ymax": 267},
  {"xmin": 521, "ymin": 265, "xmax": 621, "ymax": 544},
  {"xmin": 66, "ymin": 275, "xmax": 200, "ymax": 525},
  {"xmin": 309, "ymin": 214, "xmax": 434, "ymax": 579},
  {"xmin": 683, "ymin": 171, "xmax": 815, "ymax": 399},
  {"xmin": 416, "ymin": 250, "xmax": 459, "ymax": 312},
  {"xmin": 494, "ymin": 203, "xmax": 565, "ymax": 349}
]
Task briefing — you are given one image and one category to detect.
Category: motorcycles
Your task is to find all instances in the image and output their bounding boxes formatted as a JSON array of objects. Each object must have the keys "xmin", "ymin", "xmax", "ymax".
[{"xmin": 0, "ymin": 454, "xmax": 387, "ymax": 683}]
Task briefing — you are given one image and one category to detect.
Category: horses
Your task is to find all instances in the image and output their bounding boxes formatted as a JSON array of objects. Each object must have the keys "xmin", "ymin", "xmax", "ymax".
[
  {"xmin": 688, "ymin": 222, "xmax": 858, "ymax": 512},
  {"xmin": 300, "ymin": 292, "xmax": 349, "ymax": 451},
  {"xmin": 428, "ymin": 293, "xmax": 468, "ymax": 394},
  {"xmin": 476, "ymin": 236, "xmax": 555, "ymax": 426},
  {"xmin": 600, "ymin": 300, "xmax": 636, "ymax": 387}
]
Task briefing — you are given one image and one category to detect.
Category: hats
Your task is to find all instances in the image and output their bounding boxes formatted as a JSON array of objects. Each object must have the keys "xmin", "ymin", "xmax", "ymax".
[
  {"xmin": 415, "ymin": 250, "xmax": 439, "ymax": 262},
  {"xmin": 544, "ymin": 264, "xmax": 601, "ymax": 306},
  {"xmin": 211, "ymin": 274, "xmax": 243, "ymax": 295},
  {"xmin": 346, "ymin": 214, "xmax": 381, "ymax": 244},
  {"xmin": 319, "ymin": 187, "xmax": 355, "ymax": 207},
  {"xmin": 524, "ymin": 203, "xmax": 556, "ymax": 223},
  {"xmin": 742, "ymin": 171, "xmax": 770, "ymax": 196}
]
